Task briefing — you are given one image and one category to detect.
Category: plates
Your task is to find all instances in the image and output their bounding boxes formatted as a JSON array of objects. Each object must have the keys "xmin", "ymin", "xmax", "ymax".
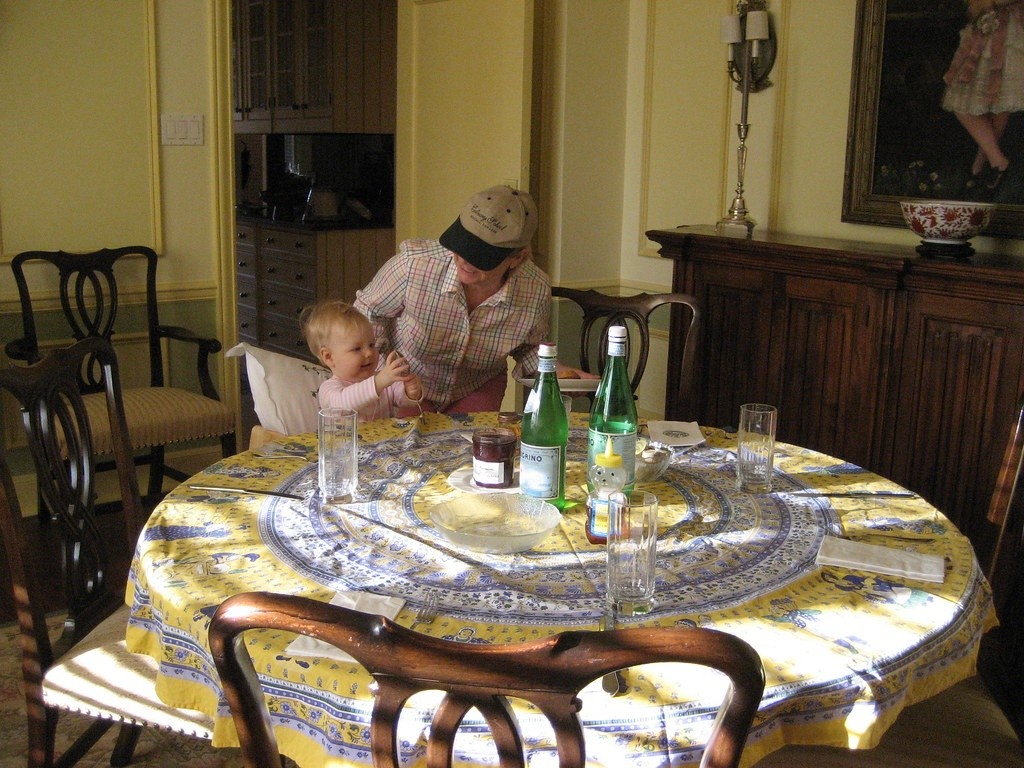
[
  {"xmin": 519, "ymin": 379, "xmax": 604, "ymax": 391},
  {"xmin": 447, "ymin": 468, "xmax": 521, "ymax": 495}
]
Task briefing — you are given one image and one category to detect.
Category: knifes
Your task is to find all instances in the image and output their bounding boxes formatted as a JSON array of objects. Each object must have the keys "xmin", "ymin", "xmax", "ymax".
[
  {"xmin": 190, "ymin": 484, "xmax": 305, "ymax": 501},
  {"xmin": 792, "ymin": 490, "xmax": 913, "ymax": 498},
  {"xmin": 823, "ymin": 491, "xmax": 914, "ymax": 496}
]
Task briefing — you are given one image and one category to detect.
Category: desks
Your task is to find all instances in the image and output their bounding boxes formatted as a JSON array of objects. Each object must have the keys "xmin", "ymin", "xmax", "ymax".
[{"xmin": 126, "ymin": 410, "xmax": 1005, "ymax": 768}]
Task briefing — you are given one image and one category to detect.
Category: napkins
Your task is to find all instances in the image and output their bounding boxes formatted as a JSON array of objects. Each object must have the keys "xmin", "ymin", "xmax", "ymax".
[
  {"xmin": 647, "ymin": 420, "xmax": 704, "ymax": 448},
  {"xmin": 815, "ymin": 534, "xmax": 948, "ymax": 585}
]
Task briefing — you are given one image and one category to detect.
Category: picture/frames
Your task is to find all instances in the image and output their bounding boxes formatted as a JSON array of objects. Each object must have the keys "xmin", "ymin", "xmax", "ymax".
[{"xmin": 842, "ymin": 0, "xmax": 1024, "ymax": 242}]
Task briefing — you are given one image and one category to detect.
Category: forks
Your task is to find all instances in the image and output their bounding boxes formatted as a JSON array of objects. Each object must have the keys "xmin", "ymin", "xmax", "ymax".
[
  {"xmin": 369, "ymin": 589, "xmax": 440, "ymax": 693},
  {"xmin": 252, "ymin": 453, "xmax": 318, "ymax": 465},
  {"xmin": 824, "ymin": 524, "xmax": 934, "ymax": 542}
]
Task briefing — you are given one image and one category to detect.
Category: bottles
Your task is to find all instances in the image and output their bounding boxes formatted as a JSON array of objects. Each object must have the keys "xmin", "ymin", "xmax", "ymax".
[
  {"xmin": 586, "ymin": 326, "xmax": 638, "ymax": 498},
  {"xmin": 497, "ymin": 412, "xmax": 522, "ymax": 469},
  {"xmin": 585, "ymin": 436, "xmax": 627, "ymax": 544},
  {"xmin": 519, "ymin": 342, "xmax": 569, "ymax": 513}
]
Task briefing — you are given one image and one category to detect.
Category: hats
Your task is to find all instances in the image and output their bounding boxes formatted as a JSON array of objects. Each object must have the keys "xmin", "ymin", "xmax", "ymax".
[{"xmin": 439, "ymin": 184, "xmax": 538, "ymax": 271}]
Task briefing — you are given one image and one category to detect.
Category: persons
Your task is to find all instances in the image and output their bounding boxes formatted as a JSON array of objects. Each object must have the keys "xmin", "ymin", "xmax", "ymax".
[
  {"xmin": 353, "ymin": 186, "xmax": 601, "ymax": 420},
  {"xmin": 304, "ymin": 302, "xmax": 425, "ymax": 424},
  {"xmin": 942, "ymin": 0, "xmax": 1024, "ymax": 191}
]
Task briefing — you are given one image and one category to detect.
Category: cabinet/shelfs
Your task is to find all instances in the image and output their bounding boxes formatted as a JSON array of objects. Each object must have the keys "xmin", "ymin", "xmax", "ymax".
[
  {"xmin": 643, "ymin": 222, "xmax": 1024, "ymax": 748},
  {"xmin": 235, "ymin": 221, "xmax": 396, "ymax": 374},
  {"xmin": 231, "ymin": 0, "xmax": 397, "ymax": 136}
]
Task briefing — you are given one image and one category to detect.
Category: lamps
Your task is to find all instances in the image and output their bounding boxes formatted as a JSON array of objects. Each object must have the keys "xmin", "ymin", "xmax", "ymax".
[{"xmin": 715, "ymin": 0, "xmax": 779, "ymax": 232}]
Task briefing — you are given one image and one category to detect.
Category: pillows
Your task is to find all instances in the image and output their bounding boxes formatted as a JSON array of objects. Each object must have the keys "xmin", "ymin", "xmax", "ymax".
[{"xmin": 222, "ymin": 337, "xmax": 334, "ymax": 437}]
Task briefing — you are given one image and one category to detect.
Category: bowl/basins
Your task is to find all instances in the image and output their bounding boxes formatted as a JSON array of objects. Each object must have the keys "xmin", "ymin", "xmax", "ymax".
[
  {"xmin": 900, "ymin": 200, "xmax": 996, "ymax": 243},
  {"xmin": 635, "ymin": 440, "xmax": 675, "ymax": 483}
]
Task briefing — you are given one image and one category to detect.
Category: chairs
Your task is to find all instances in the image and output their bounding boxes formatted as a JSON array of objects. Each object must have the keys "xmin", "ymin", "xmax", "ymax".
[
  {"xmin": 520, "ymin": 287, "xmax": 702, "ymax": 422},
  {"xmin": 751, "ymin": 397, "xmax": 1024, "ymax": 768},
  {"xmin": 3, "ymin": 246, "xmax": 238, "ymax": 523},
  {"xmin": 0, "ymin": 335, "xmax": 217, "ymax": 767},
  {"xmin": 209, "ymin": 593, "xmax": 768, "ymax": 768}
]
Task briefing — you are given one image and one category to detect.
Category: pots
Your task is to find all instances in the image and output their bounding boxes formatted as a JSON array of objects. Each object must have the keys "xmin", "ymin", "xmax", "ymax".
[{"xmin": 428, "ymin": 493, "xmax": 563, "ymax": 554}]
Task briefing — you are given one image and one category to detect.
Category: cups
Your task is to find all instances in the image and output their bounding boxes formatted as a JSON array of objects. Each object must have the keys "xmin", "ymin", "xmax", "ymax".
[
  {"xmin": 318, "ymin": 407, "xmax": 358, "ymax": 496},
  {"xmin": 562, "ymin": 394, "xmax": 571, "ymax": 417},
  {"xmin": 606, "ymin": 489, "xmax": 658, "ymax": 615},
  {"xmin": 737, "ymin": 404, "xmax": 778, "ymax": 492},
  {"xmin": 472, "ymin": 428, "xmax": 517, "ymax": 488}
]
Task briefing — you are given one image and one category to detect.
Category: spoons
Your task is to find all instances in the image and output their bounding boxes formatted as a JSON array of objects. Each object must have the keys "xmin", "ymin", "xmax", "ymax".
[{"xmin": 396, "ymin": 351, "xmax": 424, "ymax": 417}]
